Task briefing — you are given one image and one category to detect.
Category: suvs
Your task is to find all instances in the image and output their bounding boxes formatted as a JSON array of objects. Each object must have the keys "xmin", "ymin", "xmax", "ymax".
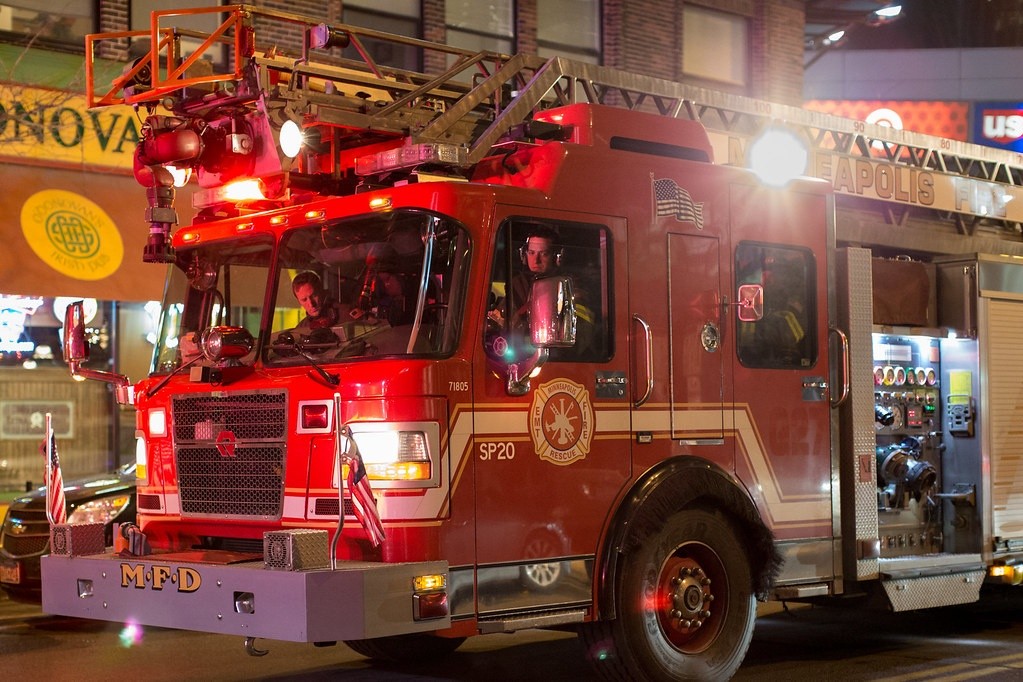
[{"xmin": 0, "ymin": 456, "xmax": 138, "ymax": 607}]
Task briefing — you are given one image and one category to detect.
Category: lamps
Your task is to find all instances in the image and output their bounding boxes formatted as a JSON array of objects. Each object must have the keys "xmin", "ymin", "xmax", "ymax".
[{"xmin": 804, "ymin": 3, "xmax": 905, "ymax": 71}]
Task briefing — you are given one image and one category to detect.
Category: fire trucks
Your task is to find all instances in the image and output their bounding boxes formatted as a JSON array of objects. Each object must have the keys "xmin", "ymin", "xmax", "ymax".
[{"xmin": 34, "ymin": 0, "xmax": 1022, "ymax": 682}]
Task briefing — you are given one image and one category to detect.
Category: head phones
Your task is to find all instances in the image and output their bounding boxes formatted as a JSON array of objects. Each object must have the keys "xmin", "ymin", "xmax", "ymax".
[{"xmin": 519, "ymin": 231, "xmax": 567, "ymax": 268}]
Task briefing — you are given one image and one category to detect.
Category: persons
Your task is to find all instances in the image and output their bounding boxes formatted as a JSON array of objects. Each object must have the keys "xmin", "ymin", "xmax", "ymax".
[
  {"xmin": 734, "ymin": 258, "xmax": 806, "ymax": 361},
  {"xmin": 237, "ymin": 271, "xmax": 338, "ymax": 358},
  {"xmin": 490, "ymin": 225, "xmax": 597, "ymax": 360}
]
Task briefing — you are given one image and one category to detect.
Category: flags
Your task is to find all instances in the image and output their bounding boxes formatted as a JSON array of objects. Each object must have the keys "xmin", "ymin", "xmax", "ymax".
[
  {"xmin": 41, "ymin": 428, "xmax": 68, "ymax": 526},
  {"xmin": 338, "ymin": 427, "xmax": 387, "ymax": 546}
]
